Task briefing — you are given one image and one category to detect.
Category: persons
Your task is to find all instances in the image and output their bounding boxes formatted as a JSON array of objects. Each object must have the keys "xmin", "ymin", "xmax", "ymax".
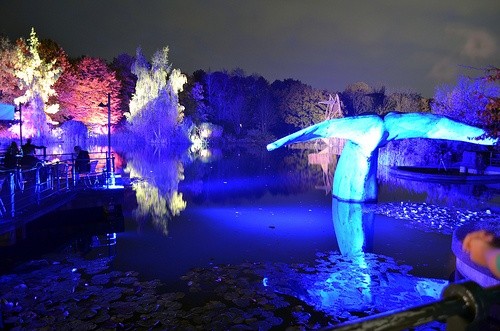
[
  {"xmin": 20, "ymin": 138, "xmax": 46, "ymax": 169},
  {"xmin": 463, "ymin": 232, "xmax": 500, "ymax": 279},
  {"xmin": 4, "ymin": 142, "xmax": 19, "ymax": 169},
  {"xmin": 74, "ymin": 146, "xmax": 91, "ymax": 173}
]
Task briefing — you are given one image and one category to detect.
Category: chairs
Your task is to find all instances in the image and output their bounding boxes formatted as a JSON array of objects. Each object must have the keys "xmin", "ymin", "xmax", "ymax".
[
  {"xmin": 49, "ymin": 162, "xmax": 68, "ymax": 191},
  {"xmin": 76, "ymin": 161, "xmax": 98, "ymax": 187}
]
